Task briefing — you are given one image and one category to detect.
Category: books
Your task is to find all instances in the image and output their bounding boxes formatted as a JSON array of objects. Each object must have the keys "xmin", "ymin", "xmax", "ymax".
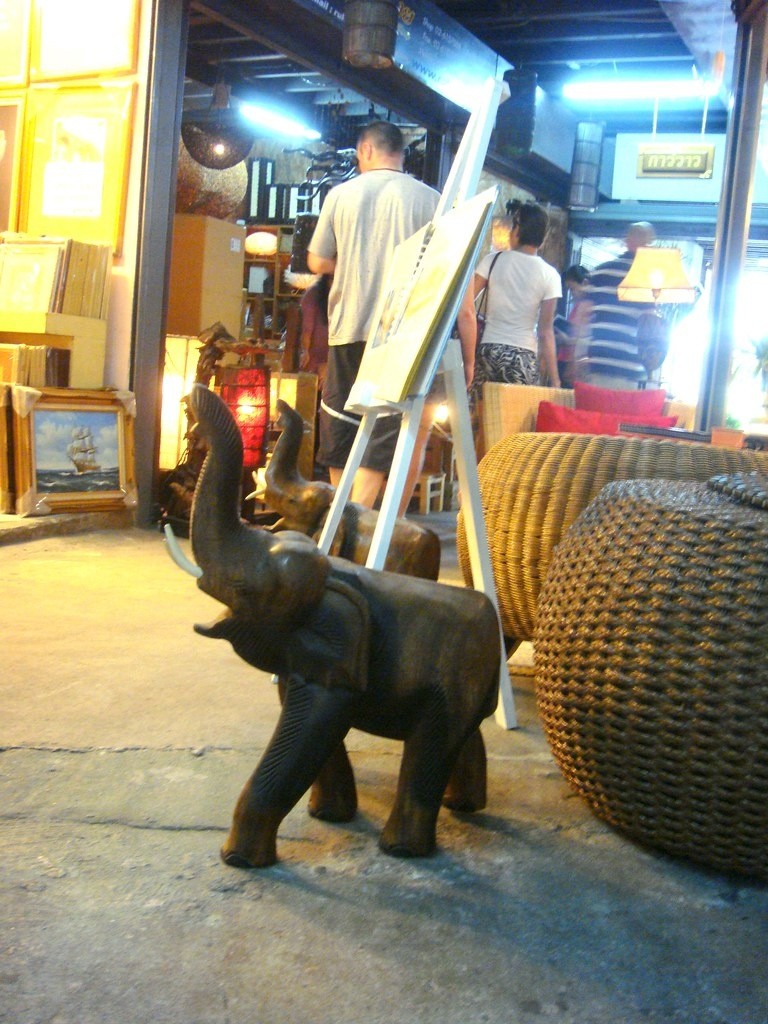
[{"xmin": 553, "ymin": 313, "xmax": 577, "ymax": 337}]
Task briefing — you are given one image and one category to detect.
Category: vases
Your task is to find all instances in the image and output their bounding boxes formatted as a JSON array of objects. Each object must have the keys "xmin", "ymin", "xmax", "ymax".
[{"xmin": 710, "ymin": 426, "xmax": 745, "ymax": 449}]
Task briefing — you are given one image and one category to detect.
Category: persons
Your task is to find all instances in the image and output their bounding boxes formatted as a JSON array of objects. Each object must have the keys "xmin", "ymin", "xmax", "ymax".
[
  {"xmin": 450, "ymin": 202, "xmax": 562, "ymax": 455},
  {"xmin": 553, "ymin": 222, "xmax": 669, "ymax": 391},
  {"xmin": 307, "ymin": 121, "xmax": 478, "ymax": 509},
  {"xmin": 299, "ymin": 273, "xmax": 334, "ymax": 396}
]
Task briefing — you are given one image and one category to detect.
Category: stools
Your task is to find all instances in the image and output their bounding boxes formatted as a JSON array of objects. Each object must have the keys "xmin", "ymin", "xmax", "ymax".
[{"xmin": 412, "ymin": 473, "xmax": 447, "ymax": 515}]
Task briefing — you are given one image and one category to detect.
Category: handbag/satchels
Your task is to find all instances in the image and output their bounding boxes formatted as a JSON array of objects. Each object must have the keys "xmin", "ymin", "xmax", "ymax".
[{"xmin": 475, "ymin": 314, "xmax": 484, "ymax": 352}]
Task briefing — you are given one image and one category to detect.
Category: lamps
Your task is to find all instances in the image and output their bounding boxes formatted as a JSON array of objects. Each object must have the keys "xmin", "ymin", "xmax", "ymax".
[{"xmin": 620, "ymin": 246, "xmax": 697, "ymax": 387}]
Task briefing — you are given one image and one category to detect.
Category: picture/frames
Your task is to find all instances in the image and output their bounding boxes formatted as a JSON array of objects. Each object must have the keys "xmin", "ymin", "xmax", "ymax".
[
  {"xmin": 0, "ymin": 0, "xmax": 143, "ymax": 259},
  {"xmin": 11, "ymin": 385, "xmax": 139, "ymax": 515}
]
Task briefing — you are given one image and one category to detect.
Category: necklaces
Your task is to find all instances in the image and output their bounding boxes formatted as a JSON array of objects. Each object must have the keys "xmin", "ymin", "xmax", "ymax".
[{"xmin": 368, "ymin": 167, "xmax": 404, "ymax": 173}]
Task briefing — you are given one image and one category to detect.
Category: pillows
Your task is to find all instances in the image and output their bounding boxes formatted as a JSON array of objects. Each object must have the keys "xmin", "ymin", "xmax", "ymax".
[
  {"xmin": 534, "ymin": 400, "xmax": 679, "ymax": 441},
  {"xmin": 574, "ymin": 381, "xmax": 666, "ymax": 417}
]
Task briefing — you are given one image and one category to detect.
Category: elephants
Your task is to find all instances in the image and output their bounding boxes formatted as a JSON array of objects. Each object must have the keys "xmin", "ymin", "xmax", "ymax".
[{"xmin": 163, "ymin": 385, "xmax": 502, "ymax": 873}]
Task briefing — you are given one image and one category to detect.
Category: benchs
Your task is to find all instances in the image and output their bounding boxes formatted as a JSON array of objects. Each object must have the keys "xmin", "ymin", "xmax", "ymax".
[{"xmin": 480, "ymin": 380, "xmax": 695, "ymax": 451}]
